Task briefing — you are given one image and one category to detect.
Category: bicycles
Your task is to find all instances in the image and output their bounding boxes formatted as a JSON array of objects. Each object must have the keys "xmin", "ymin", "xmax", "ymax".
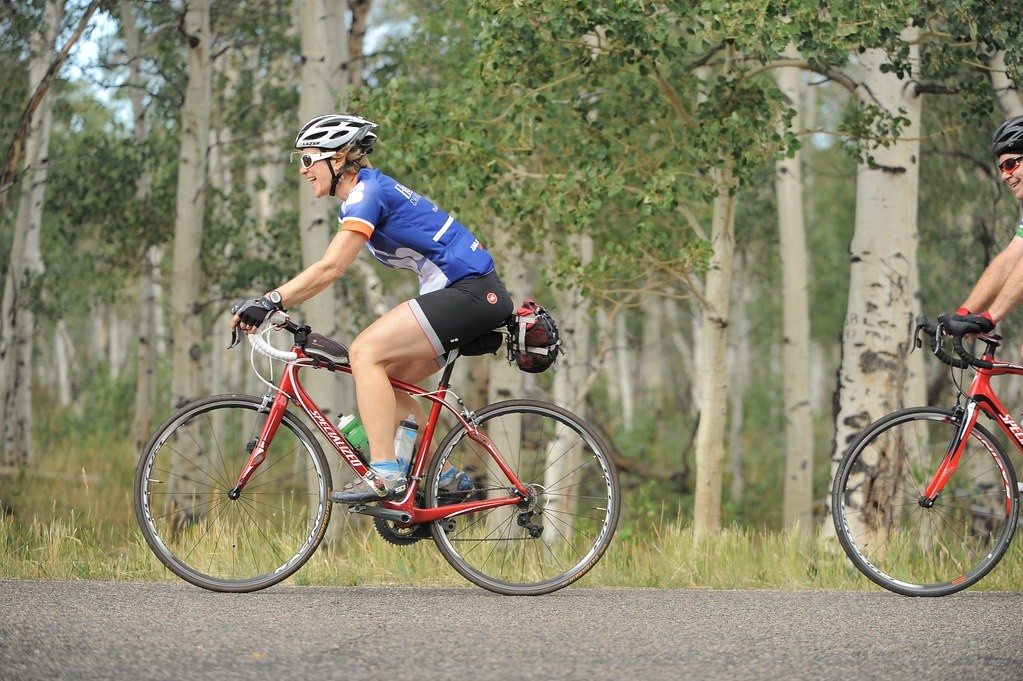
[
  {"xmin": 134, "ymin": 298, "xmax": 622, "ymax": 595},
  {"xmin": 830, "ymin": 314, "xmax": 1023, "ymax": 596}
]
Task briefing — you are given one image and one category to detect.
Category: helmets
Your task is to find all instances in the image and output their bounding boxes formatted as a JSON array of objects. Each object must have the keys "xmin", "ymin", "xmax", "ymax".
[{"xmin": 992, "ymin": 116, "xmax": 1023, "ymax": 154}]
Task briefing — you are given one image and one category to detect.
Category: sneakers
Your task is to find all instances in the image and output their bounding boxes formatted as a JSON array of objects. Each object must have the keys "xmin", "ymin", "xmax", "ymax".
[
  {"xmin": 329, "ymin": 471, "xmax": 408, "ymax": 504},
  {"xmin": 421, "ymin": 466, "xmax": 474, "ymax": 498}
]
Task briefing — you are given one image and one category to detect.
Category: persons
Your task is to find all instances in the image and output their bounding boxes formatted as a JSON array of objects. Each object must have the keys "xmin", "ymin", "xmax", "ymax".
[
  {"xmin": 933, "ymin": 113, "xmax": 1023, "ymax": 342},
  {"xmin": 229, "ymin": 107, "xmax": 517, "ymax": 521}
]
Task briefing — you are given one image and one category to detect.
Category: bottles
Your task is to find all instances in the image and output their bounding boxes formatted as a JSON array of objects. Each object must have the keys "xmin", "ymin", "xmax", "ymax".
[
  {"xmin": 336, "ymin": 413, "xmax": 371, "ymax": 464},
  {"xmin": 394, "ymin": 414, "xmax": 418, "ymax": 474}
]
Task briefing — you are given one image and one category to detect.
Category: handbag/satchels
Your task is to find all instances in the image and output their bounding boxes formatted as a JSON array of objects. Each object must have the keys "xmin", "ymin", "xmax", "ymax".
[{"xmin": 515, "ymin": 299, "xmax": 559, "ymax": 373}]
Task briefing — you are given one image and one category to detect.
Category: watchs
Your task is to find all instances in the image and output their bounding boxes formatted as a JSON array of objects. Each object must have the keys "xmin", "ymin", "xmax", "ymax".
[{"xmin": 268, "ymin": 290, "xmax": 285, "ymax": 312}]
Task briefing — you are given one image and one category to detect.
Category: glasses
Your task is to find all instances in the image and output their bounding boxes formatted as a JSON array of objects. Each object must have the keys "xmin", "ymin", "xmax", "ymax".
[
  {"xmin": 997, "ymin": 154, "xmax": 1023, "ymax": 175},
  {"xmin": 300, "ymin": 150, "xmax": 337, "ymax": 169}
]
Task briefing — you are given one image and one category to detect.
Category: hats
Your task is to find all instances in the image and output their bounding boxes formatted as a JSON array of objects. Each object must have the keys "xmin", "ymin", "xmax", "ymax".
[{"xmin": 294, "ymin": 114, "xmax": 380, "ymax": 149}]
